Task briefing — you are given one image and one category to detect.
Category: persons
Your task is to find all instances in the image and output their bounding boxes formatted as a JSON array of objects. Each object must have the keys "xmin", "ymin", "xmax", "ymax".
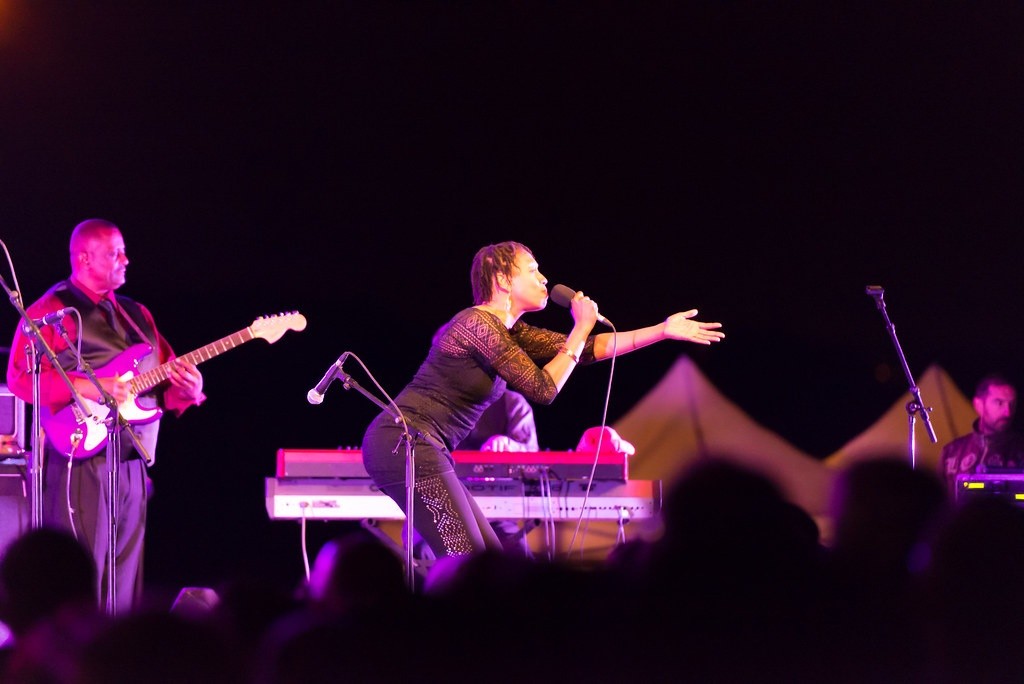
[
  {"xmin": 362, "ymin": 242, "xmax": 725, "ymax": 602},
  {"xmin": 943, "ymin": 377, "xmax": 1024, "ymax": 500},
  {"xmin": 0, "ymin": 454, "xmax": 1024, "ymax": 684},
  {"xmin": 7, "ymin": 218, "xmax": 206, "ymax": 617}
]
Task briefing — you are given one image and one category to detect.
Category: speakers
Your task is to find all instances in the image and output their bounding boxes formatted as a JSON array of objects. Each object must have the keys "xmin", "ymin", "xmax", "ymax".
[
  {"xmin": 0, "ymin": 461, "xmax": 31, "ymax": 563},
  {"xmin": 953, "ymin": 471, "xmax": 1024, "ymax": 508}
]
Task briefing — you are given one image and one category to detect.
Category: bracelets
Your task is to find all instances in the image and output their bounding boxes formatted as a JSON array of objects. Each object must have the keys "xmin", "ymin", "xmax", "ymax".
[
  {"xmin": 633, "ymin": 327, "xmax": 640, "ymax": 350},
  {"xmin": 557, "ymin": 347, "xmax": 579, "ymax": 364}
]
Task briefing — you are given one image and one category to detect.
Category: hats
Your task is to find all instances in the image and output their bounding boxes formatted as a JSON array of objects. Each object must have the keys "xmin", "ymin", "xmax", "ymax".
[{"xmin": 576, "ymin": 426, "xmax": 635, "ymax": 455}]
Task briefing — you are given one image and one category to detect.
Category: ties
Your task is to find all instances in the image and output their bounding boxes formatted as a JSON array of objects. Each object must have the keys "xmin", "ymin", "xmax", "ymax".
[{"xmin": 97, "ymin": 299, "xmax": 134, "ymax": 347}]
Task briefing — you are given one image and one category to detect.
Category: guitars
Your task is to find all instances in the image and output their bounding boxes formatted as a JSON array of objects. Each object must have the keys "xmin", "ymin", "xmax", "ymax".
[{"xmin": 40, "ymin": 311, "xmax": 306, "ymax": 459}]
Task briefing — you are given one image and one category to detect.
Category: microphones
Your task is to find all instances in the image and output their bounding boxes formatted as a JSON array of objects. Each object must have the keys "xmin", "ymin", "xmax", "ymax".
[
  {"xmin": 550, "ymin": 284, "xmax": 613, "ymax": 328},
  {"xmin": 307, "ymin": 352, "xmax": 349, "ymax": 405},
  {"xmin": 21, "ymin": 306, "xmax": 74, "ymax": 336}
]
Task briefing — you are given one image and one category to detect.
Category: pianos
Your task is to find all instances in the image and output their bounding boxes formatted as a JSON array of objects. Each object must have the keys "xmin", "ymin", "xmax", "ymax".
[{"xmin": 265, "ymin": 449, "xmax": 662, "ymax": 522}]
